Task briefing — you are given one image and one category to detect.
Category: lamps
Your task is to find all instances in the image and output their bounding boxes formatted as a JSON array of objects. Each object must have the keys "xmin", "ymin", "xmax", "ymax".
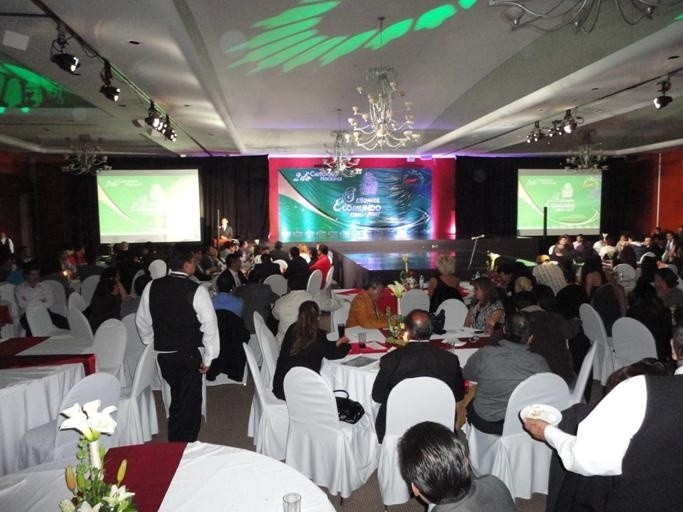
[
  {"xmin": 524, "ymin": 108, "xmax": 581, "ymax": 145},
  {"xmin": 322, "ymin": 107, "xmax": 363, "ymax": 178},
  {"xmin": 348, "ymin": 17, "xmax": 422, "ymax": 151},
  {"xmin": 62, "ymin": 134, "xmax": 111, "ymax": 176},
  {"xmin": 487, "ymin": 0, "xmax": 666, "ymax": 34},
  {"xmin": 35, "ymin": 0, "xmax": 180, "ymax": 142},
  {"xmin": 652, "ymin": 71, "xmax": 676, "ymax": 111},
  {"xmin": 563, "ymin": 130, "xmax": 609, "ymax": 172}
]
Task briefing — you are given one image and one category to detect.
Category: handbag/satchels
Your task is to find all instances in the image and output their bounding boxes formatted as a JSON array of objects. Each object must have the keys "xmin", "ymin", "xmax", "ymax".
[{"xmin": 330, "ymin": 391, "xmax": 364, "ymax": 425}]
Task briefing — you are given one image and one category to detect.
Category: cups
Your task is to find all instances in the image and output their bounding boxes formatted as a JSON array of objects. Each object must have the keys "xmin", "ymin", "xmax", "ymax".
[
  {"xmin": 359, "ymin": 332, "xmax": 367, "ymax": 349},
  {"xmin": 283, "ymin": 493, "xmax": 302, "ymax": 512}
]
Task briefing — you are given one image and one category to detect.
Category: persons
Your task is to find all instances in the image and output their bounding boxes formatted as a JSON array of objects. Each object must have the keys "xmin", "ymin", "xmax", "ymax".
[
  {"xmin": 214, "ymin": 217, "xmax": 235, "ymax": 240},
  {"xmin": 520, "ymin": 320, "xmax": 683, "ymax": 512},
  {"xmin": 395, "ymin": 424, "xmax": 516, "ymax": 512},
  {"xmin": 1, "ymin": 232, "xmax": 683, "ymax": 443}
]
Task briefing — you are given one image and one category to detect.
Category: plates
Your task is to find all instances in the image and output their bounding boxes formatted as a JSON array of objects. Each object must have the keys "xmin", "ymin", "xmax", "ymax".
[{"xmin": 518, "ymin": 403, "xmax": 565, "ymax": 427}]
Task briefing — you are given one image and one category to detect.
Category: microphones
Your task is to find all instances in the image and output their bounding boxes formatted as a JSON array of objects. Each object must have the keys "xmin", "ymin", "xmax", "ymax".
[{"xmin": 470, "ymin": 234, "xmax": 485, "ymax": 240}]
